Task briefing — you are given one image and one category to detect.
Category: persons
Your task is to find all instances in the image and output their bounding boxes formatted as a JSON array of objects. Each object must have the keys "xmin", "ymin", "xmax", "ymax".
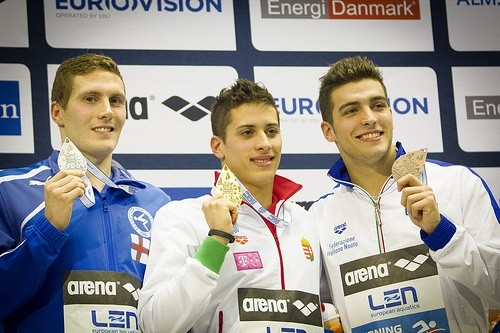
[
  {"xmin": 0, "ymin": 52, "xmax": 172, "ymax": 333},
  {"xmin": 306, "ymin": 55, "xmax": 500, "ymax": 333},
  {"xmin": 137, "ymin": 79, "xmax": 327, "ymax": 333}
]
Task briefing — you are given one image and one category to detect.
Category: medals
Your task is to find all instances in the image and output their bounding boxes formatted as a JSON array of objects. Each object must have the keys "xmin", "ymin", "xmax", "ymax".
[
  {"xmin": 391, "ymin": 147, "xmax": 428, "ymax": 181},
  {"xmin": 215, "ymin": 163, "xmax": 243, "ymax": 208},
  {"xmin": 57, "ymin": 138, "xmax": 87, "ymax": 178}
]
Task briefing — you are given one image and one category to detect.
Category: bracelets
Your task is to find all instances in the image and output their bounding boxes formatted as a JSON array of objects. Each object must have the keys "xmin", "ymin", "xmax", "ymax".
[{"xmin": 208, "ymin": 228, "xmax": 235, "ymax": 244}]
